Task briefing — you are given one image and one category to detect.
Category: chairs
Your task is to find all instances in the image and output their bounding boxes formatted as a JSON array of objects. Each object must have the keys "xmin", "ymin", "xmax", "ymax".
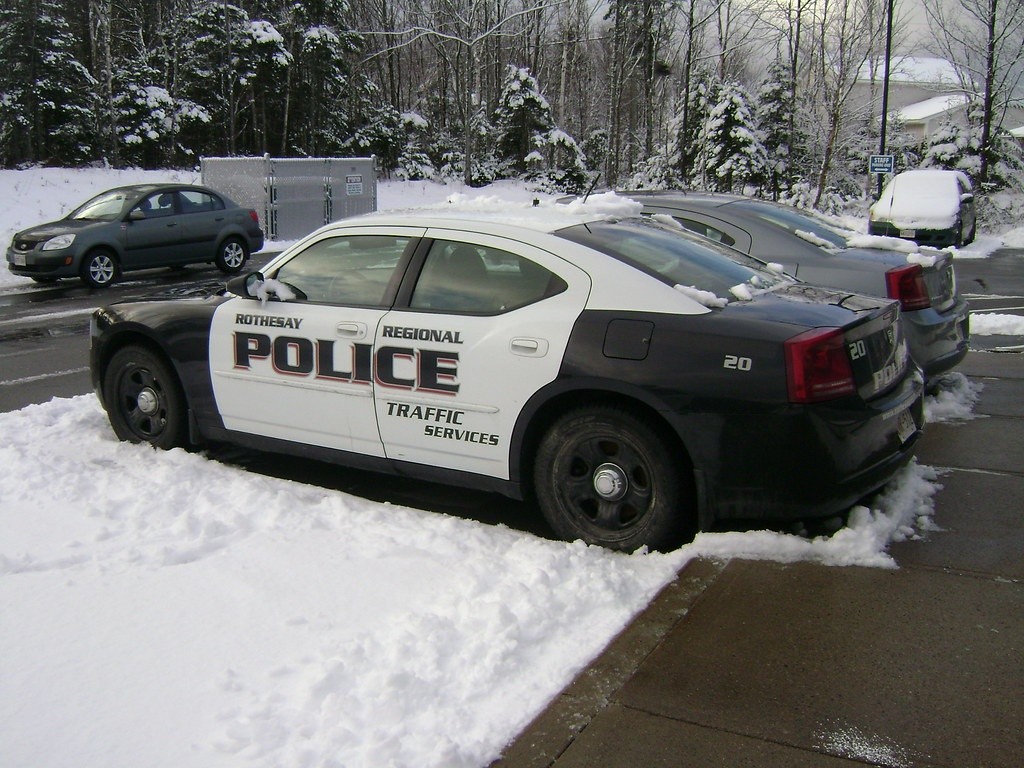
[{"xmin": 335, "ymin": 244, "xmax": 559, "ymax": 317}]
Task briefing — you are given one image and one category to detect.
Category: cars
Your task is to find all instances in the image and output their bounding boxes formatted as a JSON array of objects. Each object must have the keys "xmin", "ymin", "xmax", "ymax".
[
  {"xmin": 870, "ymin": 167, "xmax": 978, "ymax": 248},
  {"xmin": 89, "ymin": 171, "xmax": 930, "ymax": 554},
  {"xmin": 551, "ymin": 185, "xmax": 974, "ymax": 391},
  {"xmin": 6, "ymin": 182, "xmax": 267, "ymax": 292}
]
